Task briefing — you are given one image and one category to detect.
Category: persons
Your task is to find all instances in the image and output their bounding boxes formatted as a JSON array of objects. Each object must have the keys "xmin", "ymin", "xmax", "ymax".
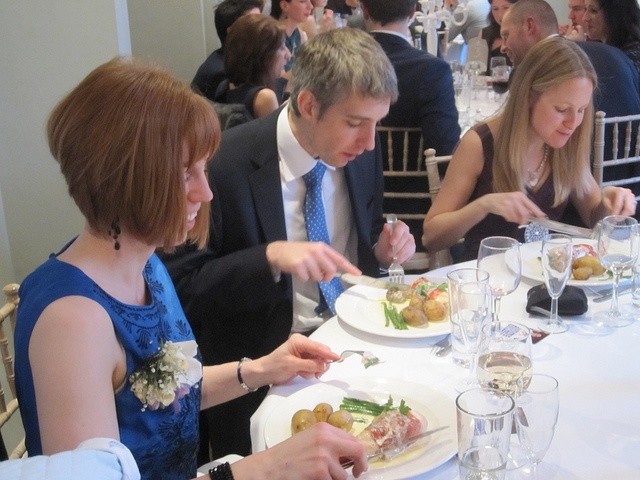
[
  {"xmin": 449, "ymin": 1, "xmax": 490, "ymax": 44},
  {"xmin": 312, "ymin": 0, "xmax": 359, "ymax": 18},
  {"xmin": 500, "ymin": 0, "xmax": 640, "ymax": 222},
  {"xmin": 558, "ymin": 0, "xmax": 586, "ymax": 40},
  {"xmin": 155, "ymin": 27, "xmax": 416, "ymax": 454},
  {"xmin": 420, "ymin": 37, "xmax": 638, "ymax": 257},
  {"xmin": 13, "ymin": 57, "xmax": 369, "ymax": 480},
  {"xmin": 583, "ymin": 0, "xmax": 640, "ymax": 70},
  {"xmin": 213, "ymin": 12, "xmax": 291, "ymax": 117},
  {"xmin": 360, "ymin": 0, "xmax": 461, "ymax": 253},
  {"xmin": 191, "ymin": 0, "xmax": 266, "ymax": 102},
  {"xmin": 268, "ymin": 0, "xmax": 313, "ymax": 98},
  {"xmin": 482, "ymin": 0, "xmax": 518, "ymax": 73}
]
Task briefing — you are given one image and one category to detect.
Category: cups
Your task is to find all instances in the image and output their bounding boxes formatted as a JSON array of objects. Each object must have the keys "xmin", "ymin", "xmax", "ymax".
[
  {"xmin": 447, "ymin": 267, "xmax": 490, "ymax": 366},
  {"xmin": 455, "ymin": 388, "xmax": 514, "ymax": 479},
  {"xmin": 629, "ymin": 224, "xmax": 640, "ymax": 307},
  {"xmin": 490, "ymin": 56, "xmax": 507, "ymax": 71}
]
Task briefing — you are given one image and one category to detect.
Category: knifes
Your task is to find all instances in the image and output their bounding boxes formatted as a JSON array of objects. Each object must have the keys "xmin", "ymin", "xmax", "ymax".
[
  {"xmin": 334, "ymin": 271, "xmax": 411, "ymax": 292},
  {"xmin": 341, "ymin": 425, "xmax": 451, "ymax": 470},
  {"xmin": 528, "ymin": 214, "xmax": 592, "ymax": 238}
]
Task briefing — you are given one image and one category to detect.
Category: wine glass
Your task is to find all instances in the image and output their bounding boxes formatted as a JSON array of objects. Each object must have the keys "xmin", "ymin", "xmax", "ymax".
[
  {"xmin": 593, "ymin": 214, "xmax": 638, "ymax": 328},
  {"xmin": 515, "ymin": 373, "xmax": 562, "ymax": 480},
  {"xmin": 476, "ymin": 234, "xmax": 522, "ymax": 341},
  {"xmin": 537, "ymin": 233, "xmax": 573, "ymax": 333},
  {"xmin": 475, "ymin": 320, "xmax": 534, "ymax": 470},
  {"xmin": 492, "ymin": 66, "xmax": 511, "ymax": 109},
  {"xmin": 455, "ymin": 82, "xmax": 472, "ymax": 127},
  {"xmin": 473, "ymin": 78, "xmax": 488, "ymax": 119},
  {"xmin": 457, "ymin": 283, "xmax": 495, "ymax": 393}
]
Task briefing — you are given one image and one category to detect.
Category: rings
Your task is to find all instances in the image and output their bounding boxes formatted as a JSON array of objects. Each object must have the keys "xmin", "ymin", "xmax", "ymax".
[{"xmin": 634, "ymin": 201, "xmax": 637, "ymax": 205}]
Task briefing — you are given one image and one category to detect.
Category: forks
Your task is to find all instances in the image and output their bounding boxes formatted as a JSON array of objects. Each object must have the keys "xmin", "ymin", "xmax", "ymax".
[
  {"xmin": 326, "ymin": 349, "xmax": 381, "ymax": 367},
  {"xmin": 386, "ymin": 213, "xmax": 405, "ymax": 285},
  {"xmin": 431, "ymin": 334, "xmax": 452, "ymax": 355}
]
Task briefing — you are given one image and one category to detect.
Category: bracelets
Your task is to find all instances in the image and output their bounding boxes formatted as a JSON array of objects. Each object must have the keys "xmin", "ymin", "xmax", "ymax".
[
  {"xmin": 208, "ymin": 462, "xmax": 235, "ymax": 480},
  {"xmin": 236, "ymin": 355, "xmax": 258, "ymax": 394}
]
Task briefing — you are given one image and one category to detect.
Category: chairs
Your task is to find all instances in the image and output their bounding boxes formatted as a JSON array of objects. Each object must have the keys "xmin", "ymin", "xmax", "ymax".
[
  {"xmin": 0, "ymin": 281, "xmax": 27, "ymax": 460},
  {"xmin": 423, "ymin": 148, "xmax": 466, "ymax": 270},
  {"xmin": 376, "ymin": 125, "xmax": 433, "ymax": 219},
  {"xmin": 594, "ymin": 110, "xmax": 639, "ymax": 202}
]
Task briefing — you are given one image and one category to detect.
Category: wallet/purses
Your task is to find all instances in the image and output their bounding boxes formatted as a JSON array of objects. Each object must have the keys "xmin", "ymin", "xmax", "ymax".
[{"xmin": 526, "ymin": 281, "xmax": 587, "ymax": 318}]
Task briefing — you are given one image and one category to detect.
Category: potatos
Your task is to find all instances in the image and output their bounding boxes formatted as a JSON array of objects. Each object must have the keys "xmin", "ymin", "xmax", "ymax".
[
  {"xmin": 291, "ymin": 409, "xmax": 317, "ymax": 437},
  {"xmin": 574, "ymin": 255, "xmax": 604, "ymax": 275},
  {"xmin": 326, "ymin": 410, "xmax": 352, "ymax": 432},
  {"xmin": 313, "ymin": 402, "xmax": 332, "ymax": 422},
  {"xmin": 422, "ymin": 300, "xmax": 444, "ymax": 320},
  {"xmin": 409, "ymin": 298, "xmax": 422, "ymax": 311},
  {"xmin": 571, "ymin": 267, "xmax": 594, "ymax": 280},
  {"xmin": 403, "ymin": 306, "xmax": 428, "ymax": 325}
]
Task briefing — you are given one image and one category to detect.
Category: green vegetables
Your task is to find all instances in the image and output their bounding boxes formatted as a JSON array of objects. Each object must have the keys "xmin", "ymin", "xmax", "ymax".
[
  {"xmin": 339, "ymin": 396, "xmax": 411, "ymax": 419},
  {"xmin": 383, "ymin": 301, "xmax": 408, "ymax": 329}
]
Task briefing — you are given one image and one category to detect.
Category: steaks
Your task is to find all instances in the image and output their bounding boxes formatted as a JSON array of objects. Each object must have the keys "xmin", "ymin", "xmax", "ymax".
[{"xmin": 550, "ymin": 244, "xmax": 597, "ymax": 271}]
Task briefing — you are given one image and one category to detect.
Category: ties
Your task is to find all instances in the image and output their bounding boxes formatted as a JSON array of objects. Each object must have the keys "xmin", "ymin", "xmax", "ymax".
[{"xmin": 302, "ymin": 162, "xmax": 343, "ymax": 316}]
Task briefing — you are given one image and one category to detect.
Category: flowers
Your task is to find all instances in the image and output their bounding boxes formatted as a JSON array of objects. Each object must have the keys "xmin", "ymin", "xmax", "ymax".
[{"xmin": 131, "ymin": 341, "xmax": 201, "ymax": 412}]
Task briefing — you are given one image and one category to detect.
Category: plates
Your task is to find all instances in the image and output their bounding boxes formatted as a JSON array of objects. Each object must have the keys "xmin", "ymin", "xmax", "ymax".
[
  {"xmin": 504, "ymin": 237, "xmax": 640, "ymax": 289},
  {"xmin": 334, "ymin": 274, "xmax": 482, "ymax": 339},
  {"xmin": 264, "ymin": 375, "xmax": 475, "ymax": 479}
]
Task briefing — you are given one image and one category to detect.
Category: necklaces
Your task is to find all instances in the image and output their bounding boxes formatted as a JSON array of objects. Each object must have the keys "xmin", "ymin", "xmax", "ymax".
[{"xmin": 527, "ymin": 144, "xmax": 550, "ymax": 189}]
